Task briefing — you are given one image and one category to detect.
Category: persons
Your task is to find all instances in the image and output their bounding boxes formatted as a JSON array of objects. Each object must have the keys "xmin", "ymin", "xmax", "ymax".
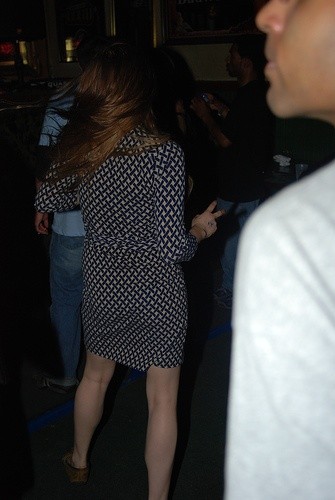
[
  {"xmin": 222, "ymin": 0, "xmax": 335, "ymax": 500},
  {"xmin": 34, "ymin": 34, "xmax": 267, "ymax": 500}
]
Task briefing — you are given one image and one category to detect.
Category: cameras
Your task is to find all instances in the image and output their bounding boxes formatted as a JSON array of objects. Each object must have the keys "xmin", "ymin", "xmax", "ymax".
[{"xmin": 201, "ymin": 95, "xmax": 208, "ymax": 103}]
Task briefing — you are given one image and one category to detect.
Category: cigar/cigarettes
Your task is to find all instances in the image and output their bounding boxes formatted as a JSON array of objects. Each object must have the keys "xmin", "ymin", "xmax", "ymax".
[{"xmin": 37, "ymin": 228, "xmax": 47, "ymax": 235}]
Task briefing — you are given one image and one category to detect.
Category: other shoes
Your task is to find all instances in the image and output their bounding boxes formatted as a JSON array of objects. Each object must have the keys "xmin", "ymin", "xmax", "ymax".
[
  {"xmin": 62, "ymin": 451, "xmax": 89, "ymax": 487},
  {"xmin": 32, "ymin": 375, "xmax": 80, "ymax": 395},
  {"xmin": 211, "ymin": 288, "xmax": 232, "ymax": 310}
]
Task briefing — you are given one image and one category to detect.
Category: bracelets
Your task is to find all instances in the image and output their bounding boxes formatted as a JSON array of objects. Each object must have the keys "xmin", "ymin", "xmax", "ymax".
[{"xmin": 191, "ymin": 225, "xmax": 207, "ymax": 239}]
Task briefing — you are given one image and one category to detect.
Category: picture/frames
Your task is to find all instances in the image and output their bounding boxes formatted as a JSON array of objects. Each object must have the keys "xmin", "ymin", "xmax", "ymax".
[{"xmin": 161, "ymin": 0, "xmax": 267, "ymax": 45}]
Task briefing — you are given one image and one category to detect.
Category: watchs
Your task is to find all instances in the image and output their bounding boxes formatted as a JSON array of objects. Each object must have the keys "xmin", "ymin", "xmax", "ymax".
[{"xmin": 217, "ymin": 106, "xmax": 227, "ymax": 116}]
